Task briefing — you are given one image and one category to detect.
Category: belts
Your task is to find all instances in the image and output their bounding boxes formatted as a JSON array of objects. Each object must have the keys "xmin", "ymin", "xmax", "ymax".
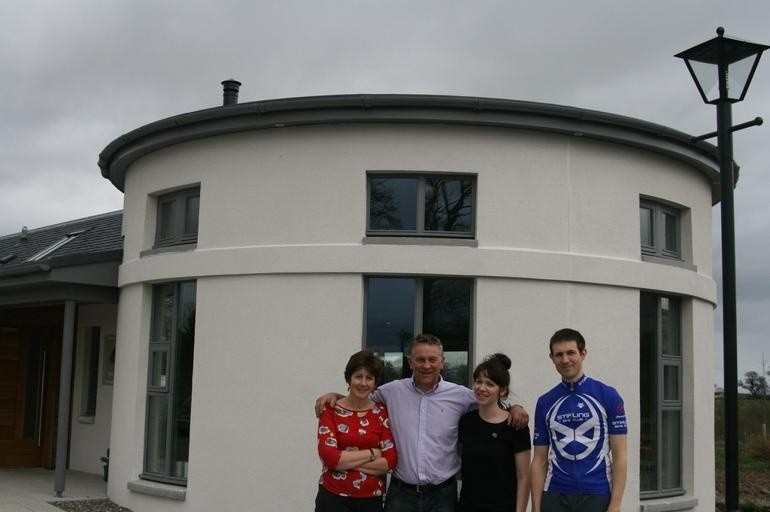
[{"xmin": 392, "ymin": 475, "xmax": 455, "ymax": 492}]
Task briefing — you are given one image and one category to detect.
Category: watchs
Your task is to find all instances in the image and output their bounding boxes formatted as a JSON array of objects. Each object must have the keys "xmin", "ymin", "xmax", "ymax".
[{"xmin": 368, "ymin": 448, "xmax": 377, "ymax": 461}]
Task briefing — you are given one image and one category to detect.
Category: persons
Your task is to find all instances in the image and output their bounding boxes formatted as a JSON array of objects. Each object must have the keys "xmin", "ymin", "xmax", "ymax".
[
  {"xmin": 315, "ymin": 350, "xmax": 398, "ymax": 512},
  {"xmin": 457, "ymin": 352, "xmax": 531, "ymax": 512},
  {"xmin": 315, "ymin": 333, "xmax": 529, "ymax": 511},
  {"xmin": 528, "ymin": 328, "xmax": 628, "ymax": 510}
]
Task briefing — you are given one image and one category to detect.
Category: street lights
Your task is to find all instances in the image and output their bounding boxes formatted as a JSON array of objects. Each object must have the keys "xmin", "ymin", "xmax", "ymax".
[{"xmin": 673, "ymin": 25, "xmax": 770, "ymax": 509}]
